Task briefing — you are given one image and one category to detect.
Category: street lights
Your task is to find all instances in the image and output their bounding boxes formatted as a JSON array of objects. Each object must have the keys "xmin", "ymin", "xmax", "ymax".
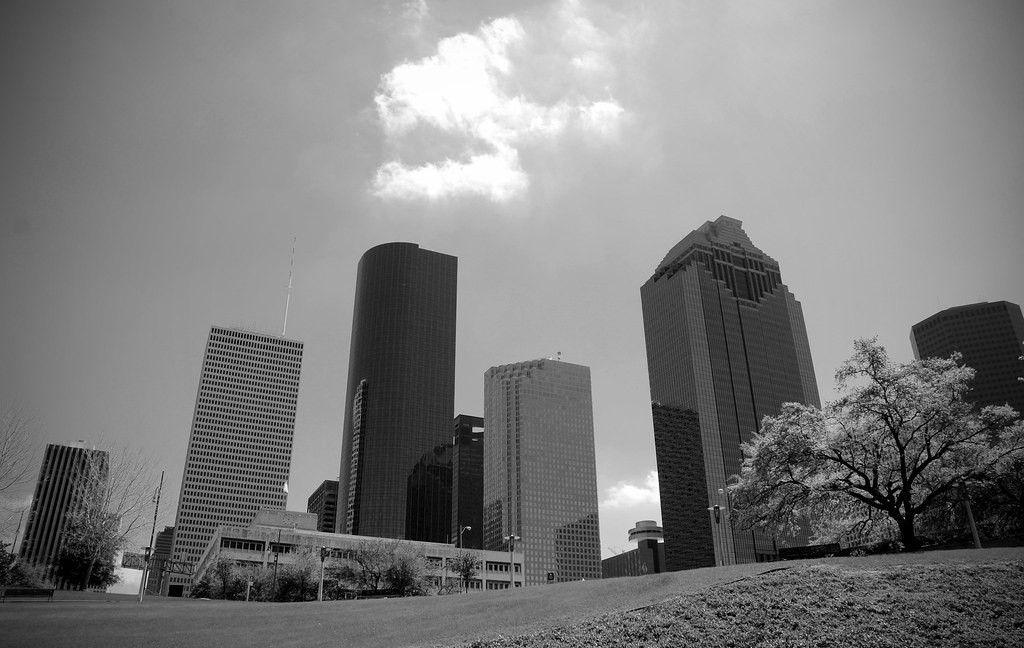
[
  {"xmin": 709, "ymin": 489, "xmax": 724, "ymax": 565},
  {"xmin": 504, "ymin": 535, "xmax": 521, "ymax": 588},
  {"xmin": 459, "ymin": 526, "xmax": 471, "ymax": 593},
  {"xmin": 139, "ymin": 487, "xmax": 161, "ymax": 602}
]
